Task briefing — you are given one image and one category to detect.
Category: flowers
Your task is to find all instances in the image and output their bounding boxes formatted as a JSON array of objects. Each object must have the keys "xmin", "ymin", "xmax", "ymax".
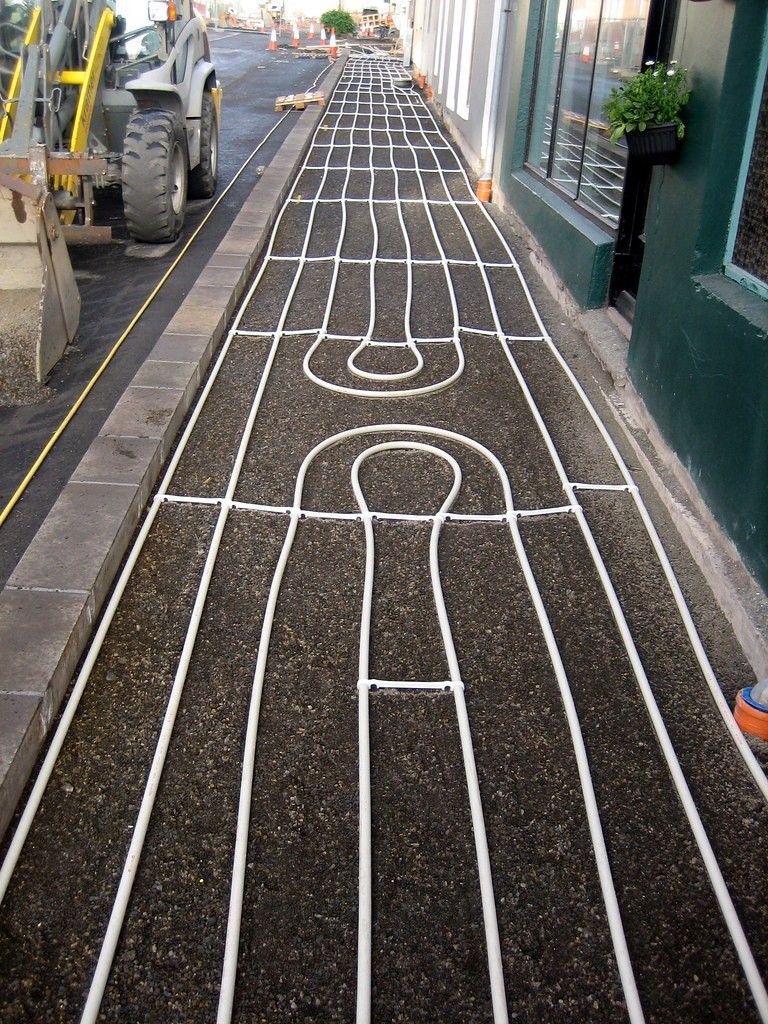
[{"xmin": 602, "ymin": 59, "xmax": 693, "ymax": 147}]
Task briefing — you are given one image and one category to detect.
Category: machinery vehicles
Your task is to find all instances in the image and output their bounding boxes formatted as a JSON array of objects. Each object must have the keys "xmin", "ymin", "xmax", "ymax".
[{"xmin": 0, "ymin": 0, "xmax": 225, "ymax": 384}]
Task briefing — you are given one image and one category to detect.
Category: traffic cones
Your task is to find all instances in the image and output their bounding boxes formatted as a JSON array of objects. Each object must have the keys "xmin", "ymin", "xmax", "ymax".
[
  {"xmin": 306, "ymin": 23, "xmax": 316, "ymax": 40},
  {"xmin": 319, "ymin": 24, "xmax": 327, "ymax": 46},
  {"xmin": 613, "ymin": 41, "xmax": 622, "ymax": 55},
  {"xmin": 290, "ymin": 29, "xmax": 300, "ymax": 49},
  {"xmin": 266, "ymin": 24, "xmax": 279, "ymax": 51},
  {"xmin": 580, "ymin": 40, "xmax": 591, "ymax": 63},
  {"xmin": 328, "ymin": 27, "xmax": 338, "ymax": 58},
  {"xmin": 361, "ymin": 25, "xmax": 366, "ymax": 37},
  {"xmin": 368, "ymin": 25, "xmax": 375, "ymax": 38},
  {"xmin": 290, "ymin": 23, "xmax": 298, "ymax": 36}
]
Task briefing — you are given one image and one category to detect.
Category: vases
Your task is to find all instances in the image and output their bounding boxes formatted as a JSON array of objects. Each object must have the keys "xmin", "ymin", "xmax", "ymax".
[{"xmin": 624, "ymin": 122, "xmax": 679, "ymax": 167}]
